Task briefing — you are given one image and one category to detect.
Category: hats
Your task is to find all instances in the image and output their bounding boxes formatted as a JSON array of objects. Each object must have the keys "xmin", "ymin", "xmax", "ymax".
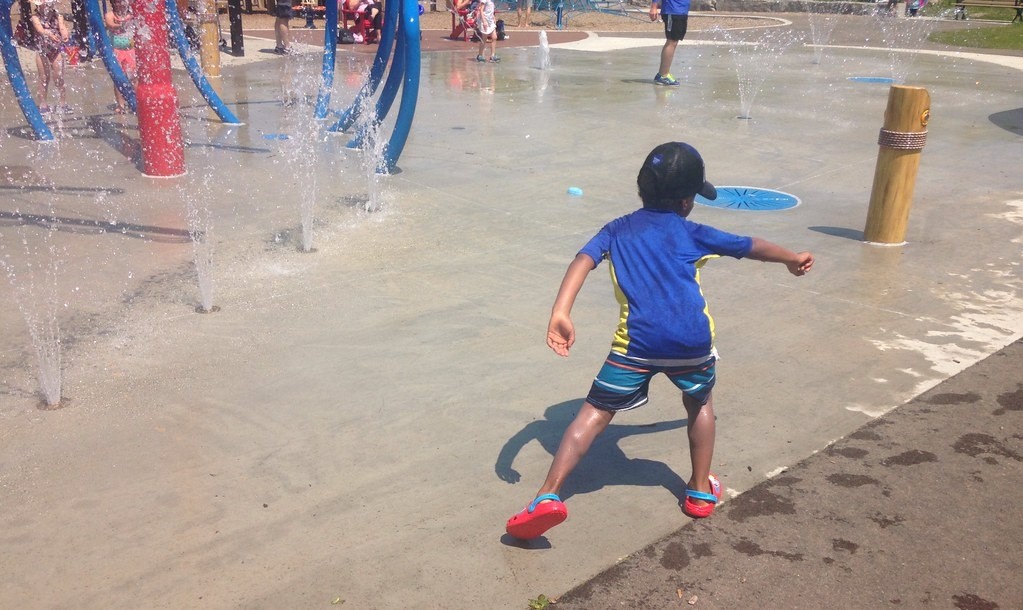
[{"xmin": 638, "ymin": 141, "xmax": 717, "ymax": 200}]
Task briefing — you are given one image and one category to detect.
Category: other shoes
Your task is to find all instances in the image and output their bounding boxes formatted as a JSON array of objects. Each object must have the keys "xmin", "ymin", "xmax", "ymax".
[
  {"xmin": 490, "ymin": 56, "xmax": 500, "ymax": 62},
  {"xmin": 654, "ymin": 73, "xmax": 661, "ymax": 81},
  {"xmin": 656, "ymin": 74, "xmax": 679, "ymax": 86},
  {"xmin": 476, "ymin": 55, "xmax": 486, "ymax": 61},
  {"xmin": 282, "ymin": 46, "xmax": 303, "ymax": 56},
  {"xmin": 274, "ymin": 45, "xmax": 284, "ymax": 54}
]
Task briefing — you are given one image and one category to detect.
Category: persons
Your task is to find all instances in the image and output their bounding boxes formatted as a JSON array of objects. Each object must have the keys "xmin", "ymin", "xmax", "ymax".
[
  {"xmin": 476, "ymin": 0, "xmax": 501, "ymax": 63},
  {"xmin": 516, "ymin": 0, "xmax": 533, "ymax": 28},
  {"xmin": 348, "ymin": 0, "xmax": 382, "ymax": 42},
  {"xmin": 30, "ymin": 0, "xmax": 138, "ymax": 118},
  {"xmin": 275, "ymin": 0, "xmax": 293, "ymax": 54},
  {"xmin": 506, "ymin": 141, "xmax": 815, "ymax": 540},
  {"xmin": 649, "ymin": 0, "xmax": 691, "ymax": 87}
]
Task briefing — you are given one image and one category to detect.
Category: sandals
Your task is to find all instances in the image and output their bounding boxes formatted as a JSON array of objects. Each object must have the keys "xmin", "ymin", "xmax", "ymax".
[
  {"xmin": 506, "ymin": 494, "xmax": 567, "ymax": 540},
  {"xmin": 684, "ymin": 473, "xmax": 721, "ymax": 517}
]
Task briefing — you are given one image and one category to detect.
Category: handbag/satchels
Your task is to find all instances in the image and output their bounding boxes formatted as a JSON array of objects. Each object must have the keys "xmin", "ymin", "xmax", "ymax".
[{"xmin": 337, "ymin": 28, "xmax": 364, "ymax": 43}]
